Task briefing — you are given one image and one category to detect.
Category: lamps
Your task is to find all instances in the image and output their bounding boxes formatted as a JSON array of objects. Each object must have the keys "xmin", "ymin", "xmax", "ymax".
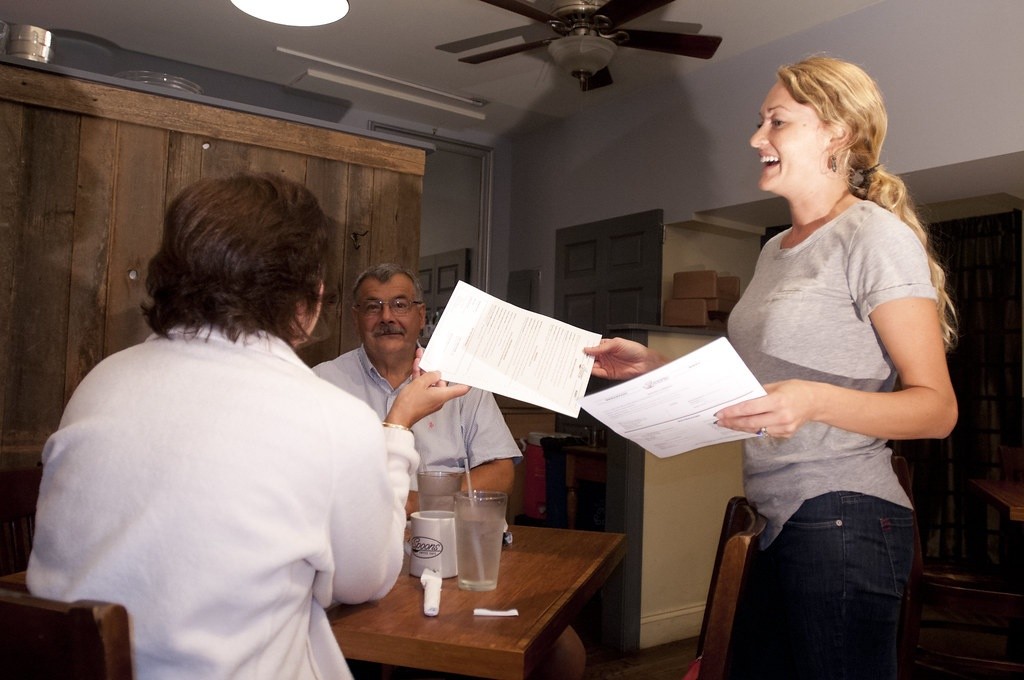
[{"xmin": 547, "ymin": 34, "xmax": 618, "ymax": 81}]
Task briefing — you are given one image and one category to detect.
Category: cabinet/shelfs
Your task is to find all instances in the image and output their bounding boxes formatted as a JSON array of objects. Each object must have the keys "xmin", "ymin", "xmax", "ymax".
[{"xmin": 0, "ymin": 53, "xmax": 435, "ymax": 470}]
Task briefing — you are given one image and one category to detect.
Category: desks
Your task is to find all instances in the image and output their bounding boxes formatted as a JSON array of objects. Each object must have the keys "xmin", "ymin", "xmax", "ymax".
[
  {"xmin": 319, "ymin": 524, "xmax": 625, "ymax": 680},
  {"xmin": 967, "ymin": 477, "xmax": 1024, "ymax": 680},
  {"xmin": 563, "ymin": 444, "xmax": 608, "ymax": 530}
]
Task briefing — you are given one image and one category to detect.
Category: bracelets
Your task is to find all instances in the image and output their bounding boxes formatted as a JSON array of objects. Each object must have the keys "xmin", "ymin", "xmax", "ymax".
[{"xmin": 382, "ymin": 422, "xmax": 414, "ymax": 434}]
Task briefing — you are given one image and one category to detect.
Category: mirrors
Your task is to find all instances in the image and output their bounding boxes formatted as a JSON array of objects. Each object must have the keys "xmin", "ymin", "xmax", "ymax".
[{"xmin": 367, "ymin": 120, "xmax": 495, "ymax": 348}]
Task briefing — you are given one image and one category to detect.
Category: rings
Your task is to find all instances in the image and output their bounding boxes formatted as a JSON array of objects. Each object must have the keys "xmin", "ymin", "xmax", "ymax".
[{"xmin": 756, "ymin": 427, "xmax": 768, "ymax": 438}]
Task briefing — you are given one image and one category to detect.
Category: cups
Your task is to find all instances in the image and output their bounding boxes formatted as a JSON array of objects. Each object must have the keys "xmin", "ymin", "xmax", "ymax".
[
  {"xmin": 403, "ymin": 511, "xmax": 458, "ymax": 579},
  {"xmin": 417, "ymin": 471, "xmax": 465, "ymax": 514},
  {"xmin": 0, "ymin": 21, "xmax": 57, "ymax": 65},
  {"xmin": 579, "ymin": 428, "xmax": 605, "ymax": 447},
  {"xmin": 454, "ymin": 490, "xmax": 508, "ymax": 592}
]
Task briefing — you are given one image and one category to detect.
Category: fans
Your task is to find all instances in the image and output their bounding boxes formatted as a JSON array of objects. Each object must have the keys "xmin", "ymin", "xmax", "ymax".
[{"xmin": 457, "ymin": 0, "xmax": 723, "ymax": 91}]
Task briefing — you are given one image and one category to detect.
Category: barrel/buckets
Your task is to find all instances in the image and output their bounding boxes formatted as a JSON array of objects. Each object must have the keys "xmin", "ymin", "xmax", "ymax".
[{"xmin": 522, "ymin": 430, "xmax": 582, "ymax": 518}]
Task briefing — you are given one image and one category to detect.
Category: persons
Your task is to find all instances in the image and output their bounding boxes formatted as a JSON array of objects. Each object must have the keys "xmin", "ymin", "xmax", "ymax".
[
  {"xmin": 28, "ymin": 172, "xmax": 472, "ymax": 680},
  {"xmin": 311, "ymin": 262, "xmax": 584, "ymax": 680},
  {"xmin": 584, "ymin": 55, "xmax": 959, "ymax": 680}
]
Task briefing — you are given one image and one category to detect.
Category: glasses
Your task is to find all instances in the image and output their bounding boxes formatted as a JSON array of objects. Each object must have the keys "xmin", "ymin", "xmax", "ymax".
[{"xmin": 352, "ymin": 298, "xmax": 422, "ymax": 314}]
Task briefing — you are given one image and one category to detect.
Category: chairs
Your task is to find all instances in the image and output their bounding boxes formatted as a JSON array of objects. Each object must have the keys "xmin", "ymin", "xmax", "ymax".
[
  {"xmin": 694, "ymin": 496, "xmax": 769, "ymax": 680},
  {"xmin": 0, "ymin": 588, "xmax": 134, "ymax": 680},
  {"xmin": 0, "ymin": 470, "xmax": 44, "ymax": 578},
  {"xmin": 885, "ymin": 446, "xmax": 1024, "ymax": 680}
]
